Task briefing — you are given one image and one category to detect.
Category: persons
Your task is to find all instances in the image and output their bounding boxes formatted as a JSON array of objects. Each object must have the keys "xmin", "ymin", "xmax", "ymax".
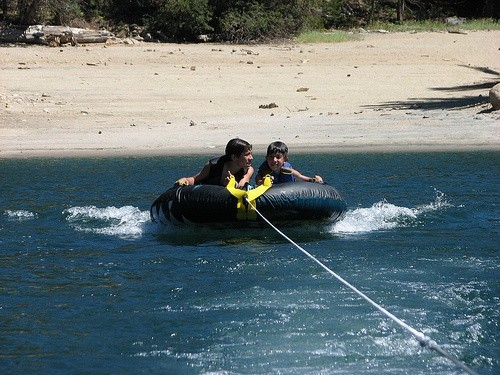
[
  {"xmin": 255, "ymin": 141, "xmax": 324, "ymax": 186},
  {"xmin": 175, "ymin": 138, "xmax": 254, "ymax": 189}
]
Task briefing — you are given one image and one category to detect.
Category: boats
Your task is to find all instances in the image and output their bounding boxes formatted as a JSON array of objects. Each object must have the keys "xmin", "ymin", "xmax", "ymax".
[{"xmin": 151, "ymin": 171, "xmax": 348, "ymax": 225}]
